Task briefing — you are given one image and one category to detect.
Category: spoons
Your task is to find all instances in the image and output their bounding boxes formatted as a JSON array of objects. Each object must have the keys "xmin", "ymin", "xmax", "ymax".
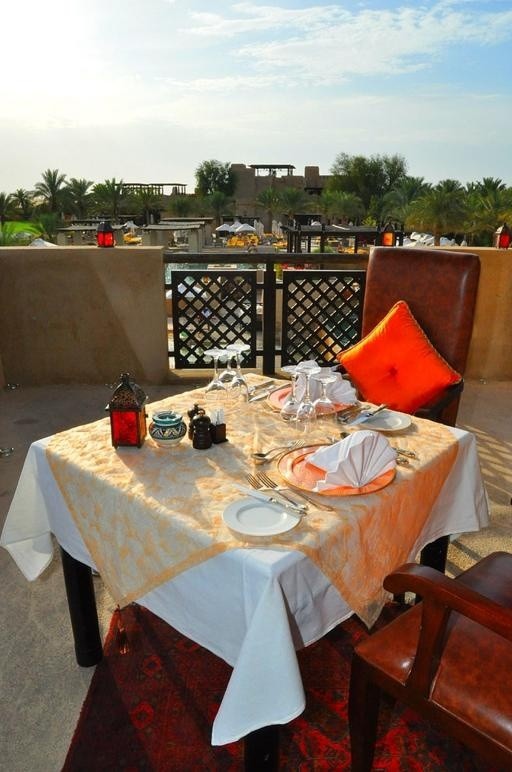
[
  {"xmin": 250, "ymin": 440, "xmax": 293, "ymax": 456},
  {"xmin": 337, "ymin": 431, "xmax": 415, "ymax": 458}
]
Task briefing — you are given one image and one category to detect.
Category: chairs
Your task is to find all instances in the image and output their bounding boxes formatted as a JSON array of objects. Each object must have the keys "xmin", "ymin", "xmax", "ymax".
[
  {"xmin": 332, "ymin": 246, "xmax": 480, "ymax": 604},
  {"xmin": 348, "ymin": 551, "xmax": 512, "ymax": 772}
]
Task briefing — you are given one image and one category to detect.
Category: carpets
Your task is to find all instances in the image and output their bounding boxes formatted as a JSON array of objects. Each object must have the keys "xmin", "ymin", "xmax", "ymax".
[{"xmin": 61, "ymin": 600, "xmax": 495, "ymax": 772}]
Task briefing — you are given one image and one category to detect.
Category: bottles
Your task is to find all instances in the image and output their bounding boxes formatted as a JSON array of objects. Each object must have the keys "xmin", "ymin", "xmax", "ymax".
[
  {"xmin": 192, "ymin": 415, "xmax": 211, "ymax": 449},
  {"xmin": 188, "ymin": 403, "xmax": 205, "ymax": 440}
]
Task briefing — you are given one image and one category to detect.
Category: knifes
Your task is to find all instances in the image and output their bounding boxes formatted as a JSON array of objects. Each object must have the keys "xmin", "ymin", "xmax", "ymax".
[
  {"xmin": 250, "ymin": 382, "xmax": 290, "ymax": 404},
  {"xmin": 230, "ymin": 482, "xmax": 306, "ymax": 515},
  {"xmin": 344, "ymin": 403, "xmax": 386, "ymax": 428}
]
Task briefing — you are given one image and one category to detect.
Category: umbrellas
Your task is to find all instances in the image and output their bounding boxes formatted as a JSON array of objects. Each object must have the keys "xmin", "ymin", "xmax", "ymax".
[
  {"xmin": 230, "ymin": 222, "xmax": 241, "ymax": 232},
  {"xmin": 216, "ymin": 224, "xmax": 230, "ymax": 237},
  {"xmin": 235, "ymin": 223, "xmax": 256, "ymax": 233}
]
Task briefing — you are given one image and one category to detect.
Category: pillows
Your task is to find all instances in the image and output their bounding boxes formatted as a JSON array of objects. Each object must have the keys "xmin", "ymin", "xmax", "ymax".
[{"xmin": 337, "ymin": 300, "xmax": 465, "ymax": 416}]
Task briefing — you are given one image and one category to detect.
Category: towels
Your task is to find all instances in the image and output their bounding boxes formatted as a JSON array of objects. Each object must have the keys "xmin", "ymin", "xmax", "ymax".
[
  {"xmin": 283, "ymin": 359, "xmax": 357, "ymax": 408},
  {"xmin": 305, "ymin": 429, "xmax": 398, "ymax": 492}
]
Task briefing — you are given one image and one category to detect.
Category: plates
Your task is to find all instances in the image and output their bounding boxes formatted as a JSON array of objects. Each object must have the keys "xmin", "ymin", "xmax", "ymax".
[
  {"xmin": 357, "ymin": 410, "xmax": 412, "ymax": 431},
  {"xmin": 224, "ymin": 497, "xmax": 300, "ymax": 536},
  {"xmin": 268, "ymin": 386, "xmax": 355, "ymax": 417},
  {"xmin": 276, "ymin": 443, "xmax": 396, "ymax": 495}
]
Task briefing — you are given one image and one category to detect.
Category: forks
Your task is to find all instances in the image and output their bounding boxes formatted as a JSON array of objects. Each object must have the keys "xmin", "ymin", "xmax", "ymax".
[
  {"xmin": 245, "ymin": 473, "xmax": 300, "ymax": 492},
  {"xmin": 260, "ymin": 401, "xmax": 279, "ymax": 417},
  {"xmin": 255, "ymin": 473, "xmax": 309, "ymax": 512},
  {"xmin": 268, "ymin": 438, "xmax": 306, "ymax": 463}
]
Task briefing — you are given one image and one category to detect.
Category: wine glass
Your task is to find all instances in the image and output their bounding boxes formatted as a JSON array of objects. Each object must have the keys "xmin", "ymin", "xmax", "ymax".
[
  {"xmin": 310, "ymin": 374, "xmax": 337, "ymax": 434},
  {"xmin": 296, "ymin": 368, "xmax": 320, "ymax": 432},
  {"xmin": 204, "ymin": 350, "xmax": 227, "ymax": 412},
  {"xmin": 219, "ymin": 350, "xmax": 241, "ymax": 399},
  {"xmin": 227, "ymin": 343, "xmax": 251, "ymax": 412},
  {"xmin": 279, "ymin": 365, "xmax": 300, "ymax": 432}
]
